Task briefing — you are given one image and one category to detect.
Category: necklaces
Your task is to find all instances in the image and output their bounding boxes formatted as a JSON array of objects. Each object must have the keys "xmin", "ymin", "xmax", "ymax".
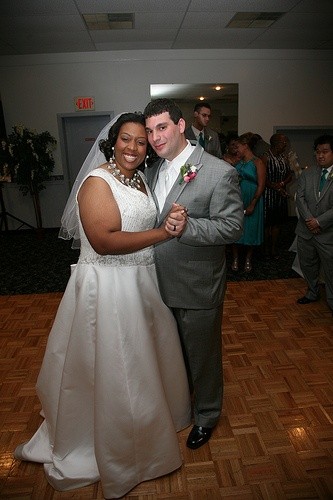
[{"xmin": 108, "ymin": 157, "xmax": 141, "ymax": 190}]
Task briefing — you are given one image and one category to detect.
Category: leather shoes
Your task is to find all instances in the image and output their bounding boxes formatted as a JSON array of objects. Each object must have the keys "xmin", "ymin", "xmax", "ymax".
[
  {"xmin": 297, "ymin": 296, "xmax": 321, "ymax": 304},
  {"xmin": 186, "ymin": 425, "xmax": 216, "ymax": 450}
]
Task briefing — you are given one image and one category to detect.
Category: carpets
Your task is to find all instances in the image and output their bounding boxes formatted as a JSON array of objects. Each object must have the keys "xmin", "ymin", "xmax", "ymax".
[{"xmin": 0, "ymin": 227, "xmax": 301, "ymax": 296}]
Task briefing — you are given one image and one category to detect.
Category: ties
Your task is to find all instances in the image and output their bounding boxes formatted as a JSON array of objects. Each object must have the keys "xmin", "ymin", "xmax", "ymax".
[
  {"xmin": 198, "ymin": 131, "xmax": 205, "ymax": 151},
  {"xmin": 319, "ymin": 168, "xmax": 328, "ymax": 192}
]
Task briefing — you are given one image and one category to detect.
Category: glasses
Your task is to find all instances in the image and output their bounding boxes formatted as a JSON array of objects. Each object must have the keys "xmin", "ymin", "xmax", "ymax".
[
  {"xmin": 196, "ymin": 111, "xmax": 212, "ymax": 119},
  {"xmin": 314, "ymin": 150, "xmax": 331, "ymax": 156}
]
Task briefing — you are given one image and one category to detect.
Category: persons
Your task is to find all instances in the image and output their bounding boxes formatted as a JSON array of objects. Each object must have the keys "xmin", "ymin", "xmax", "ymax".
[
  {"xmin": 184, "ymin": 103, "xmax": 222, "ymax": 159},
  {"xmin": 143, "ymin": 98, "xmax": 245, "ymax": 449},
  {"xmin": 223, "ymin": 132, "xmax": 296, "ymax": 273},
  {"xmin": 294, "ymin": 135, "xmax": 333, "ymax": 312},
  {"xmin": 13, "ymin": 112, "xmax": 193, "ymax": 500}
]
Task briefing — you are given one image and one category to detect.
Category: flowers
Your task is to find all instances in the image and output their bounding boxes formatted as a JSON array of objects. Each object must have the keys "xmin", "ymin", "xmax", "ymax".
[
  {"xmin": 178, "ymin": 163, "xmax": 198, "ymax": 185},
  {"xmin": 0, "ymin": 123, "xmax": 58, "ymax": 198}
]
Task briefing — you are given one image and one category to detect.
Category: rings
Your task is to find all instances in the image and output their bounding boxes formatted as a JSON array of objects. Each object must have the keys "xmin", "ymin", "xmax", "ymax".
[{"xmin": 173, "ymin": 225, "xmax": 176, "ymax": 231}]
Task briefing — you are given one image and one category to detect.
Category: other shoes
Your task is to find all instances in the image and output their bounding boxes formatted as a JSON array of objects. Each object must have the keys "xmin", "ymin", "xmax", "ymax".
[
  {"xmin": 244, "ymin": 258, "xmax": 253, "ymax": 273},
  {"xmin": 231, "ymin": 257, "xmax": 240, "ymax": 272}
]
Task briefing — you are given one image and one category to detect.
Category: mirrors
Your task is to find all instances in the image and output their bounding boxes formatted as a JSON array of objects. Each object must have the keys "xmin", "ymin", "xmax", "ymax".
[{"xmin": 150, "ymin": 83, "xmax": 239, "ymax": 156}]
